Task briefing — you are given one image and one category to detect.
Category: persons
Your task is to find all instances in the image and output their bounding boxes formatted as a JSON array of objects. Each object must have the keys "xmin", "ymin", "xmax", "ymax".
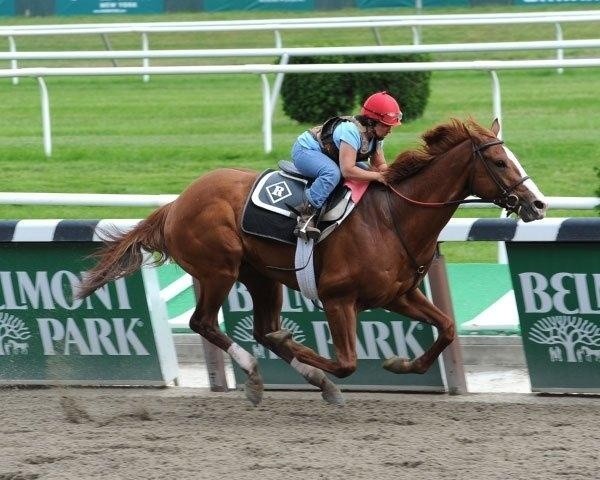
[{"xmin": 292, "ymin": 91, "xmax": 403, "ymax": 239}]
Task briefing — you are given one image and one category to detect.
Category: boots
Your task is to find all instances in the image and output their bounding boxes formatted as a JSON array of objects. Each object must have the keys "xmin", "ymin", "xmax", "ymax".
[{"xmin": 293, "ymin": 204, "xmax": 321, "ymax": 239}]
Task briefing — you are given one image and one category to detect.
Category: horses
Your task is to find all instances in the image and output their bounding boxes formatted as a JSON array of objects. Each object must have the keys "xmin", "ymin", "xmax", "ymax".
[{"xmin": 67, "ymin": 112, "xmax": 549, "ymax": 407}]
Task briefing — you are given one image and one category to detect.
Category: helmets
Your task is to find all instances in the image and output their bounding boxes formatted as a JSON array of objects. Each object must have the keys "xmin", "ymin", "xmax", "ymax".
[{"xmin": 362, "ymin": 92, "xmax": 401, "ymax": 126}]
